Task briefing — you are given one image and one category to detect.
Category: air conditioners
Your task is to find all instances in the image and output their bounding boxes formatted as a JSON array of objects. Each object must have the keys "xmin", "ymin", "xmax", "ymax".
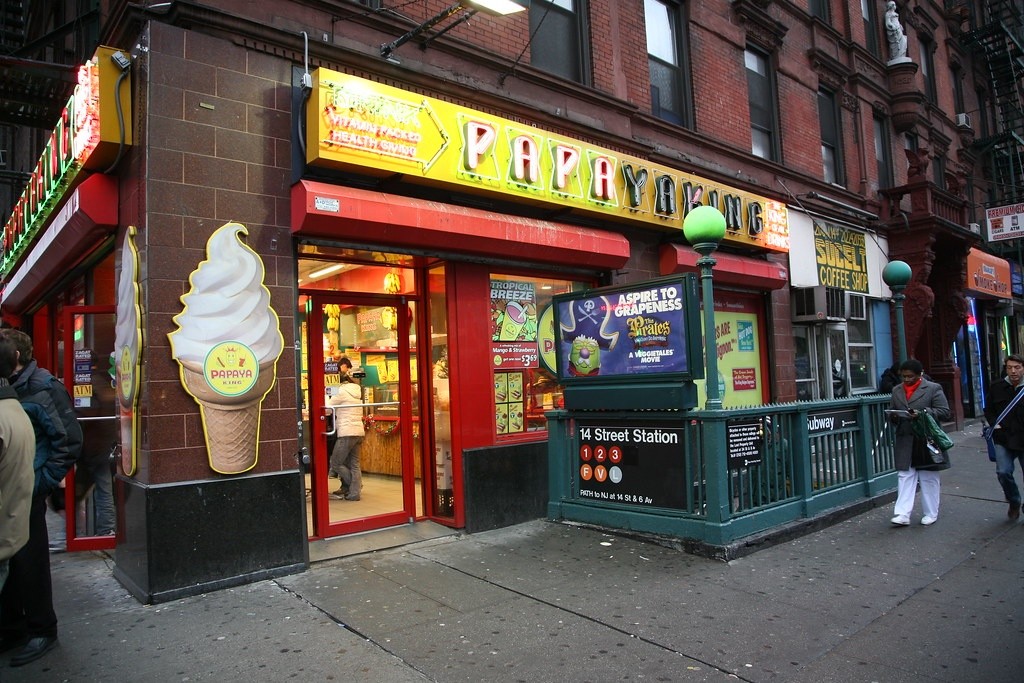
[
  {"xmin": 969, "ymin": 222, "xmax": 980, "ymax": 233},
  {"xmin": 955, "ymin": 112, "xmax": 971, "ymax": 128}
]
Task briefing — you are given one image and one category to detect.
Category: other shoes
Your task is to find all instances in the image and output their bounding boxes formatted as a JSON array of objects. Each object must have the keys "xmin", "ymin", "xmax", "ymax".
[
  {"xmin": 8, "ymin": 636, "xmax": 60, "ymax": 667},
  {"xmin": 921, "ymin": 515, "xmax": 938, "ymax": 525},
  {"xmin": 332, "ymin": 485, "xmax": 345, "ymax": 495},
  {"xmin": 329, "ymin": 468, "xmax": 338, "ymax": 478},
  {"xmin": 890, "ymin": 516, "xmax": 910, "ymax": 526},
  {"xmin": 1008, "ymin": 497, "xmax": 1021, "ymax": 519}
]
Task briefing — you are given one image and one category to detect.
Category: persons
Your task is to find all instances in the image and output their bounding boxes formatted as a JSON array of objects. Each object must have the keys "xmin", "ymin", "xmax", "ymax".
[
  {"xmin": 48, "ymin": 348, "xmax": 117, "ymax": 552},
  {"xmin": 889, "ymin": 360, "xmax": 950, "ymax": 525},
  {"xmin": 983, "ymin": 354, "xmax": 1024, "ymax": 519},
  {"xmin": 326, "ymin": 357, "xmax": 365, "ymax": 500},
  {"xmin": 0, "ymin": 334, "xmax": 36, "ymax": 588},
  {"xmin": 0, "ymin": 328, "xmax": 84, "ymax": 667}
]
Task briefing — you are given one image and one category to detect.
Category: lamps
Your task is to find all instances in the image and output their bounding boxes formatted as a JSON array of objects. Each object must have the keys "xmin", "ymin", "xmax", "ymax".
[{"xmin": 379, "ymin": 0, "xmax": 531, "ymax": 58}]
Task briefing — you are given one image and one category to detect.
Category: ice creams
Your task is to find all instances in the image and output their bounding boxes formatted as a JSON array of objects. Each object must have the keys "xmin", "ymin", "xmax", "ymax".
[{"xmin": 168, "ymin": 223, "xmax": 285, "ymax": 475}]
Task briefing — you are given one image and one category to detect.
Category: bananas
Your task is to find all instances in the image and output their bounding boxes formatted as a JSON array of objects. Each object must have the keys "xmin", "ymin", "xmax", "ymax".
[
  {"xmin": 325, "ymin": 303, "xmax": 340, "ymax": 330},
  {"xmin": 383, "ymin": 273, "xmax": 400, "ymax": 293}
]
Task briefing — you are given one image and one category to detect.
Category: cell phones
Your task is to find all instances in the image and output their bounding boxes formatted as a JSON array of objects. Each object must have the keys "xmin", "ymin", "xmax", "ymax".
[{"xmin": 909, "ymin": 409, "xmax": 915, "ymax": 413}]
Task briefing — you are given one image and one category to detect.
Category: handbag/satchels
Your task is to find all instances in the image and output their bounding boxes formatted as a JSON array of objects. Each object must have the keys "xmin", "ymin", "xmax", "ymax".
[
  {"xmin": 910, "ymin": 409, "xmax": 954, "ymax": 452},
  {"xmin": 980, "ymin": 425, "xmax": 997, "ymax": 462}
]
